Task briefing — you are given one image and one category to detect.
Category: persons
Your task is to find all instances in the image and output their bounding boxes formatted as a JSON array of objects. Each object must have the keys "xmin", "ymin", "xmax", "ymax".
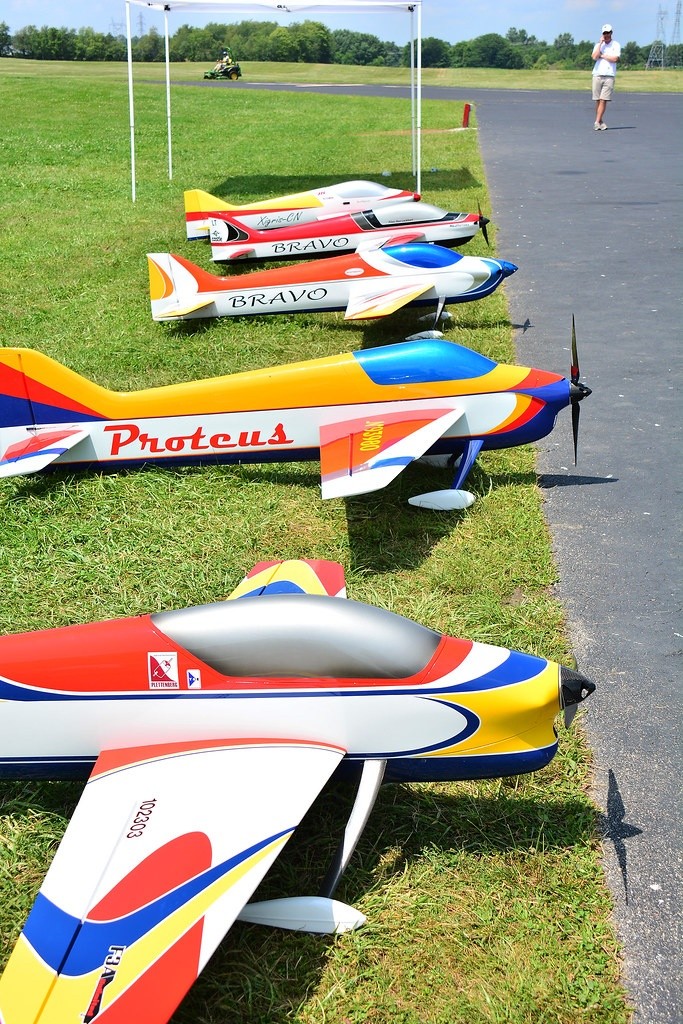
[
  {"xmin": 213, "ymin": 51, "xmax": 229, "ymax": 71},
  {"xmin": 591, "ymin": 24, "xmax": 621, "ymax": 131}
]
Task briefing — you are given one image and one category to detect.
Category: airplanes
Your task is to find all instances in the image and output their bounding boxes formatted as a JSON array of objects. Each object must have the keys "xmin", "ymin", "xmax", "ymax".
[
  {"xmin": 146, "ymin": 244, "xmax": 519, "ymax": 342},
  {"xmin": 210, "ymin": 200, "xmax": 491, "ymax": 265},
  {"xmin": 184, "ymin": 180, "xmax": 422, "ymax": 240},
  {"xmin": 0, "ymin": 316, "xmax": 592, "ymax": 508},
  {"xmin": 0, "ymin": 560, "xmax": 596, "ymax": 1024}
]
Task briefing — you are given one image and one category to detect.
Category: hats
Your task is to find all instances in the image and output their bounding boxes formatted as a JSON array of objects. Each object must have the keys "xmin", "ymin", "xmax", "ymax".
[
  {"xmin": 223, "ymin": 51, "xmax": 226, "ymax": 54},
  {"xmin": 602, "ymin": 24, "xmax": 613, "ymax": 33}
]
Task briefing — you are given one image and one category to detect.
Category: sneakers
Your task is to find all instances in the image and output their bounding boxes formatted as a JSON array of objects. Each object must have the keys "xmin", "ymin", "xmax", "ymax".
[
  {"xmin": 593, "ymin": 123, "xmax": 601, "ymax": 130},
  {"xmin": 601, "ymin": 122, "xmax": 608, "ymax": 131}
]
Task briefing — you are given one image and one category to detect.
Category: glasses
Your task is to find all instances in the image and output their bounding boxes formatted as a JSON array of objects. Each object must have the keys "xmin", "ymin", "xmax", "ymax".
[
  {"xmin": 603, "ymin": 31, "xmax": 612, "ymax": 35},
  {"xmin": 223, "ymin": 54, "xmax": 226, "ymax": 55}
]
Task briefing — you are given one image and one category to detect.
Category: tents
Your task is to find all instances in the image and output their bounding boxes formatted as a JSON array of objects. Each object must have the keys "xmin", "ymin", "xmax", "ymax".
[{"xmin": 125, "ymin": 0, "xmax": 423, "ymax": 200}]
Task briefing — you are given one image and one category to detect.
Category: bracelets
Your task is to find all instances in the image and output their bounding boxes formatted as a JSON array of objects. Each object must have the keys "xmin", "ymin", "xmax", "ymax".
[
  {"xmin": 600, "ymin": 54, "xmax": 603, "ymax": 58},
  {"xmin": 599, "ymin": 40, "xmax": 602, "ymax": 43}
]
